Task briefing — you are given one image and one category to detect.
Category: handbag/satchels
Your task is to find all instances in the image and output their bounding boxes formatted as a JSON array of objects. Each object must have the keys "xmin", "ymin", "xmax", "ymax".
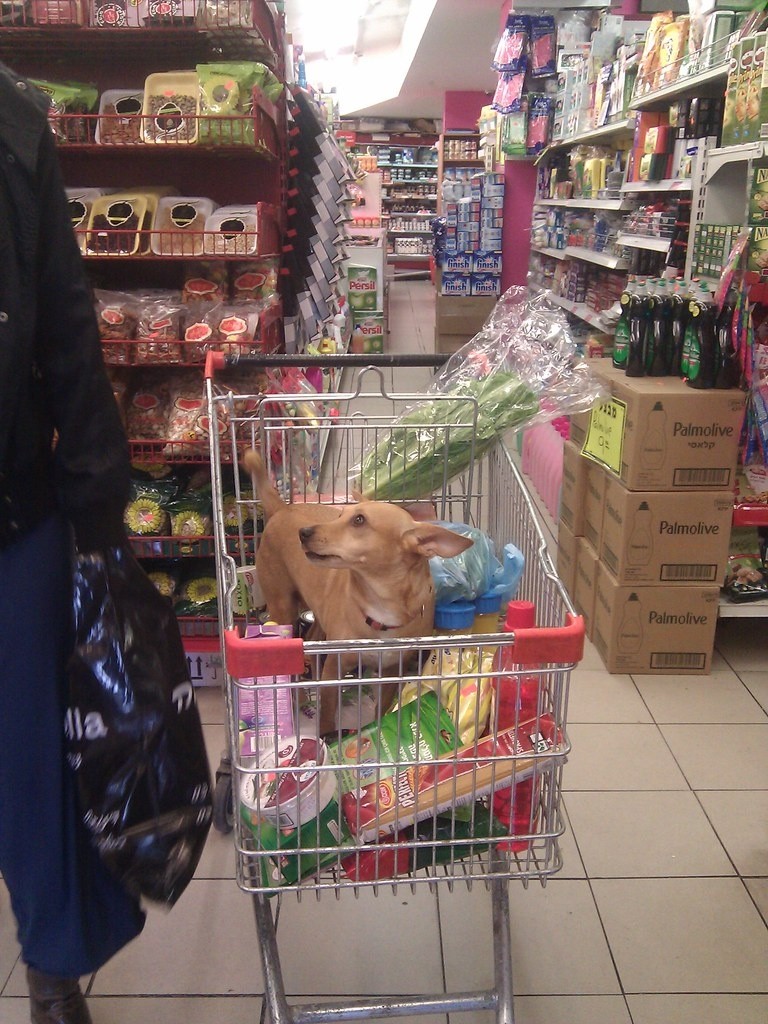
[{"xmin": 66, "ymin": 521, "xmax": 213, "ymax": 911}]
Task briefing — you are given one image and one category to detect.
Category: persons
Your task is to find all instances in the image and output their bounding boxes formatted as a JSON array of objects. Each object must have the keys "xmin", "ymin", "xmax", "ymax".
[{"xmin": 0, "ymin": 61, "xmax": 147, "ymax": 1024}]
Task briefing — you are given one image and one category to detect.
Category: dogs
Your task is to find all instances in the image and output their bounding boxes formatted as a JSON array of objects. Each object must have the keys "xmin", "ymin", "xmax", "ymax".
[{"xmin": 242, "ymin": 446, "xmax": 475, "ymax": 746}]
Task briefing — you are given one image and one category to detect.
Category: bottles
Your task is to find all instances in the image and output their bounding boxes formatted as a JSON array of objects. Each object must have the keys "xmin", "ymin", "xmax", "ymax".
[
  {"xmin": 491, "ymin": 600, "xmax": 545, "ymax": 853},
  {"xmin": 612, "ymin": 276, "xmax": 738, "ymax": 390}
]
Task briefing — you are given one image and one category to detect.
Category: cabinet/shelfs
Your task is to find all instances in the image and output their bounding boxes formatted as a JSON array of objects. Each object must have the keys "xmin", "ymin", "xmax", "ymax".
[
  {"xmin": 528, "ymin": 30, "xmax": 768, "ymax": 618},
  {"xmin": 1, "ymin": 0, "xmax": 487, "ymax": 687}
]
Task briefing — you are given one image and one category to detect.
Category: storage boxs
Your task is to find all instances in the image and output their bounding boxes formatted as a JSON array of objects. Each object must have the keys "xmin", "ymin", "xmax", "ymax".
[
  {"xmin": 557, "ymin": 359, "xmax": 745, "ymax": 675},
  {"xmin": 239, "ymin": 625, "xmax": 295, "ymax": 768},
  {"xmin": 636, "ymin": 98, "xmax": 724, "ymax": 181}
]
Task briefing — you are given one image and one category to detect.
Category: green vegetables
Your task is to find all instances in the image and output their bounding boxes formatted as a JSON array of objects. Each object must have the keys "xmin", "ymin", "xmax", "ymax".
[{"xmin": 351, "ymin": 373, "xmax": 540, "ymax": 501}]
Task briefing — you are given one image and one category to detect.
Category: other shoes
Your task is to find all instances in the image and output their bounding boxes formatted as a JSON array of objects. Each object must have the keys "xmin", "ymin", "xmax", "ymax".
[{"xmin": 26, "ymin": 967, "xmax": 92, "ymax": 1024}]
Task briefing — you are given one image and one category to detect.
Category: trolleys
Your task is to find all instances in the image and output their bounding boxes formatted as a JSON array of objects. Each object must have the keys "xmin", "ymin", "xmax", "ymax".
[{"xmin": 204, "ymin": 348, "xmax": 587, "ymax": 1023}]
